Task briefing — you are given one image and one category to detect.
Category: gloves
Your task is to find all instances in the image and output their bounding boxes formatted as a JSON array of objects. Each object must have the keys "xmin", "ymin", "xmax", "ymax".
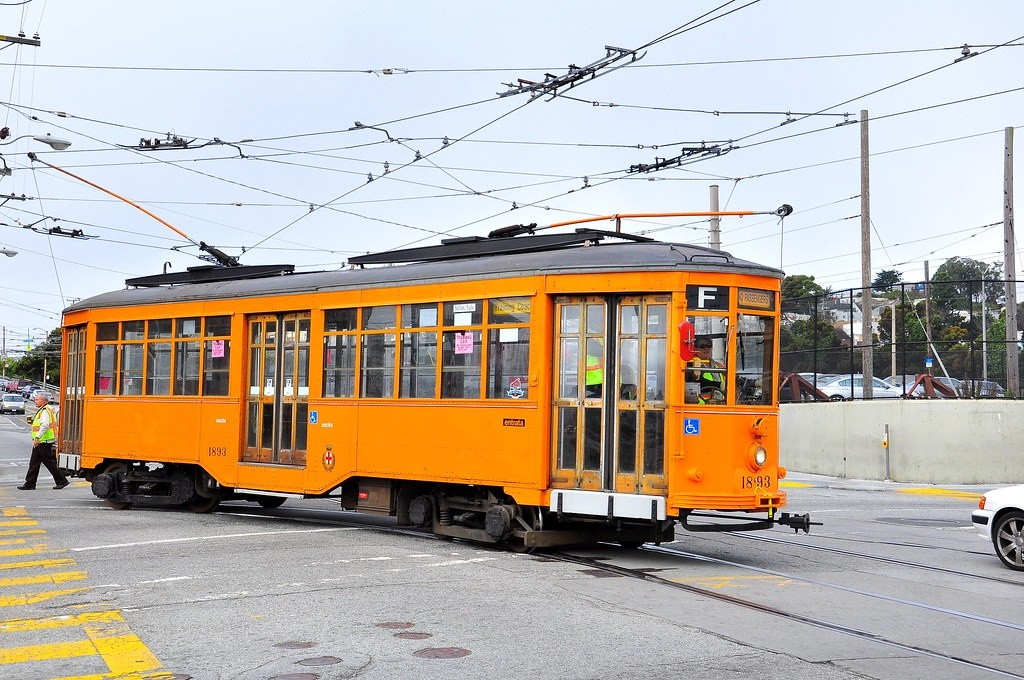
[
  {"xmin": 720, "ymin": 382, "xmax": 725, "ymax": 390},
  {"xmin": 755, "ymin": 380, "xmax": 762, "ymax": 388}
]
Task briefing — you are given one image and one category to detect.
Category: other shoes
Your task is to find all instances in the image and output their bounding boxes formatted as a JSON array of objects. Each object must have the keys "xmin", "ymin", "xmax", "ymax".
[
  {"xmin": 53, "ymin": 482, "xmax": 69, "ymax": 489},
  {"xmin": 17, "ymin": 485, "xmax": 36, "ymax": 490}
]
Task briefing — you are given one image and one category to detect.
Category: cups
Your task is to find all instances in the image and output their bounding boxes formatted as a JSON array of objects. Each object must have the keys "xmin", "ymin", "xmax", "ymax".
[{"xmin": 27, "ymin": 416, "xmax": 32, "ymax": 422}]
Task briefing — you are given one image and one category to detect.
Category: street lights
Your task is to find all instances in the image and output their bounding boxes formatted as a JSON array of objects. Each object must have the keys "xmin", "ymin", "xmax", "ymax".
[{"xmin": 33, "ymin": 328, "xmax": 49, "ymax": 343}]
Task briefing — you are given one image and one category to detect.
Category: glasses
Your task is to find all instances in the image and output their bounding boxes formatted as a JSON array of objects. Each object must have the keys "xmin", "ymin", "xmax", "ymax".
[{"xmin": 695, "ymin": 343, "xmax": 712, "ymax": 349}]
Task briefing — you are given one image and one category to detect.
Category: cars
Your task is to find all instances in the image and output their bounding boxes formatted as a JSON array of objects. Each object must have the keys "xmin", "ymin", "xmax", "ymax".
[
  {"xmin": 754, "ymin": 371, "xmax": 1009, "ymax": 405},
  {"xmin": 0, "ymin": 378, "xmax": 55, "ymax": 405},
  {"xmin": 971, "ymin": 484, "xmax": 1024, "ymax": 573},
  {"xmin": 0, "ymin": 393, "xmax": 28, "ymax": 415}
]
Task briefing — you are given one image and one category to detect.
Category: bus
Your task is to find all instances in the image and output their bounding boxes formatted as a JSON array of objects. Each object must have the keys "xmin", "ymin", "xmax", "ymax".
[{"xmin": 52, "ymin": 221, "xmax": 821, "ymax": 558}]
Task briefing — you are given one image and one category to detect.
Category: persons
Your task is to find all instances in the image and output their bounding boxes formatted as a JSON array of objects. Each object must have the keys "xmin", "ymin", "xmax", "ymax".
[
  {"xmin": 685, "ymin": 337, "xmax": 770, "ymax": 405},
  {"xmin": 581, "ymin": 317, "xmax": 643, "ymax": 416},
  {"xmin": 17, "ymin": 393, "xmax": 70, "ymax": 491}
]
